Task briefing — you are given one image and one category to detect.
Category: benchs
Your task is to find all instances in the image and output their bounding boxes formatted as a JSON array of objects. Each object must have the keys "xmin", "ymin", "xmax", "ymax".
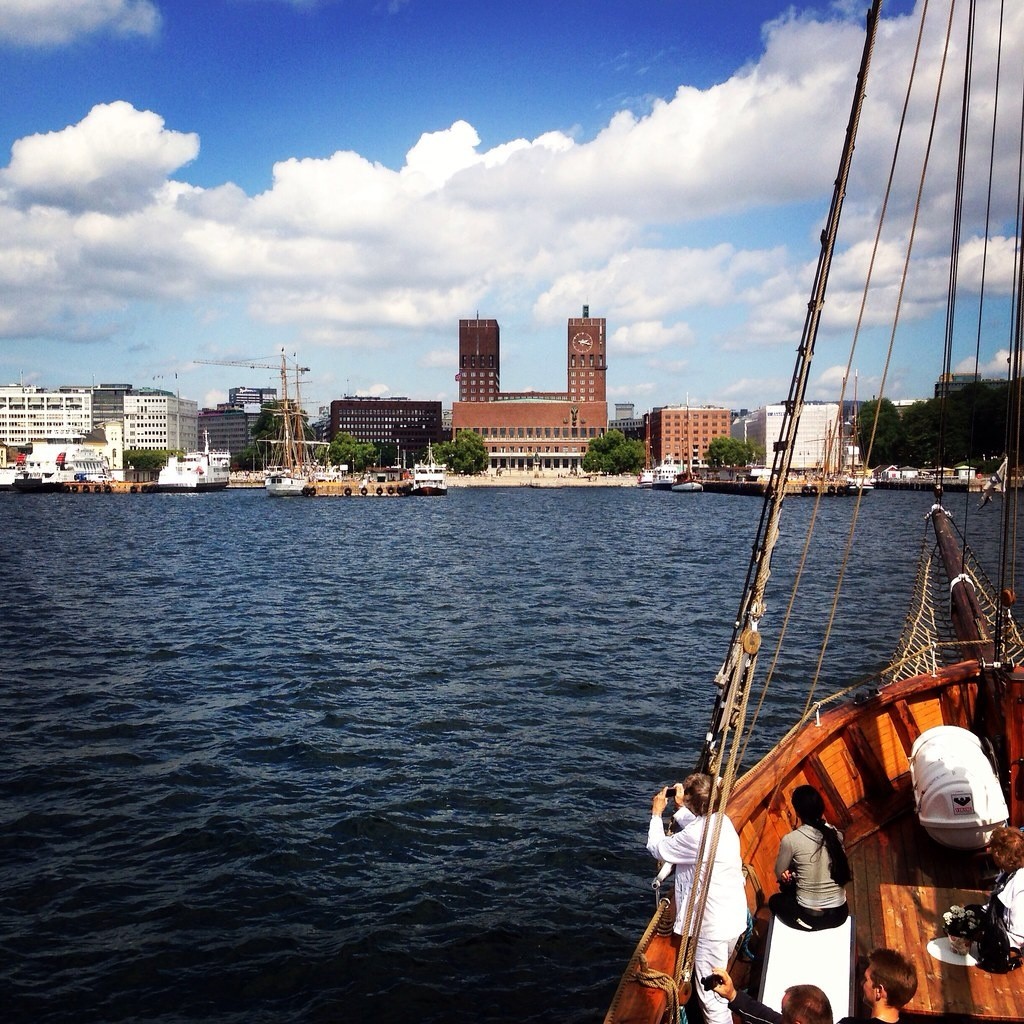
[{"xmin": 757, "ymin": 914, "xmax": 856, "ymax": 1024}]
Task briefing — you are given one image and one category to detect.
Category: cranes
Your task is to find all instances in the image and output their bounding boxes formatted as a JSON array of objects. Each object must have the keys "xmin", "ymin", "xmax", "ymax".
[{"xmin": 193, "ymin": 347, "xmax": 311, "ymax": 400}]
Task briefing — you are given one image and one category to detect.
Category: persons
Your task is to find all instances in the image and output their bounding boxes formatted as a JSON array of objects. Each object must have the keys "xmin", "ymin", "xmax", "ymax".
[
  {"xmin": 952, "ymin": 826, "xmax": 1024, "ymax": 963},
  {"xmin": 836, "ymin": 948, "xmax": 918, "ymax": 1024},
  {"xmin": 711, "ymin": 968, "xmax": 833, "ymax": 1024},
  {"xmin": 768, "ymin": 785, "xmax": 854, "ymax": 932},
  {"xmin": 646, "ymin": 774, "xmax": 748, "ymax": 1024}
]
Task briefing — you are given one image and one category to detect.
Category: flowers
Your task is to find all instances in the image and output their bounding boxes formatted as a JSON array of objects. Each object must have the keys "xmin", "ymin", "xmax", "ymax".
[{"xmin": 942, "ymin": 904, "xmax": 978, "ymax": 937}]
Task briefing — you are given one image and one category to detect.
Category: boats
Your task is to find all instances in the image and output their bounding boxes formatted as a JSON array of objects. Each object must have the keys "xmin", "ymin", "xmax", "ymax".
[
  {"xmin": 13, "ymin": 429, "xmax": 112, "ymax": 494},
  {"xmin": 155, "ymin": 427, "xmax": 233, "ymax": 493},
  {"xmin": 411, "ymin": 437, "xmax": 448, "ymax": 496},
  {"xmin": 636, "ymin": 464, "xmax": 677, "ymax": 492}
]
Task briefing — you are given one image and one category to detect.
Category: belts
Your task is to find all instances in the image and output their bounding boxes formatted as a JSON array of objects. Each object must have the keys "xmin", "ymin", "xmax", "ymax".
[{"xmin": 802, "ymin": 907, "xmax": 826, "ymax": 918}]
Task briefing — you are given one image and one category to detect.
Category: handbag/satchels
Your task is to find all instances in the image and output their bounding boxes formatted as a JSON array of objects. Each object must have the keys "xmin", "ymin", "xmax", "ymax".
[{"xmin": 964, "ymin": 893, "xmax": 1022, "ymax": 974}]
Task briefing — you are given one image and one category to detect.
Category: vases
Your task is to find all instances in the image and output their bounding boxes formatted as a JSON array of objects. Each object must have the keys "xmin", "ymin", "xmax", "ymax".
[{"xmin": 947, "ymin": 935, "xmax": 973, "ymax": 956}]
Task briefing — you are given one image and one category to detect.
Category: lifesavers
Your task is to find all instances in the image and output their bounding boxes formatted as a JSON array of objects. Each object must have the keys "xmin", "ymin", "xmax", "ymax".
[
  {"xmin": 344, "ymin": 488, "xmax": 352, "ymax": 495},
  {"xmin": 388, "ymin": 487, "xmax": 394, "ymax": 494},
  {"xmin": 376, "ymin": 488, "xmax": 383, "ymax": 494},
  {"xmin": 397, "ymin": 486, "xmax": 410, "ymax": 493},
  {"xmin": 302, "ymin": 486, "xmax": 316, "ymax": 496},
  {"xmin": 361, "ymin": 488, "xmax": 367, "ymax": 495}
]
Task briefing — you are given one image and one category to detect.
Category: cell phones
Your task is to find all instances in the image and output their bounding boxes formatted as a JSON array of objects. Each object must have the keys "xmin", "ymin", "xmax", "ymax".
[{"xmin": 665, "ymin": 787, "xmax": 676, "ymax": 797}]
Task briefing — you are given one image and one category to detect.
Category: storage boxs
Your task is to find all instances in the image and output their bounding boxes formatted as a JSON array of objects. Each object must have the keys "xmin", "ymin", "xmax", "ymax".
[{"xmin": 907, "ymin": 726, "xmax": 1009, "ymax": 850}]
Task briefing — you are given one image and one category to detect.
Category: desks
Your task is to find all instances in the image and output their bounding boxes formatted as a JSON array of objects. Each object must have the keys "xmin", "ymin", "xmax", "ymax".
[{"xmin": 880, "ymin": 883, "xmax": 1024, "ymax": 1023}]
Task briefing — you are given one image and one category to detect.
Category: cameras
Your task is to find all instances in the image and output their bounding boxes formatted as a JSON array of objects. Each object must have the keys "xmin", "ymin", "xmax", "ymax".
[{"xmin": 701, "ymin": 974, "xmax": 723, "ymax": 991}]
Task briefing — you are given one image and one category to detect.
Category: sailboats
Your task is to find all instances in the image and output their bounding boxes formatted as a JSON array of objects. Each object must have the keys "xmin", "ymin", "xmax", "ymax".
[
  {"xmin": 263, "ymin": 357, "xmax": 313, "ymax": 495},
  {"xmin": 806, "ymin": 369, "xmax": 876, "ymax": 496},
  {"xmin": 671, "ymin": 390, "xmax": 705, "ymax": 491}
]
